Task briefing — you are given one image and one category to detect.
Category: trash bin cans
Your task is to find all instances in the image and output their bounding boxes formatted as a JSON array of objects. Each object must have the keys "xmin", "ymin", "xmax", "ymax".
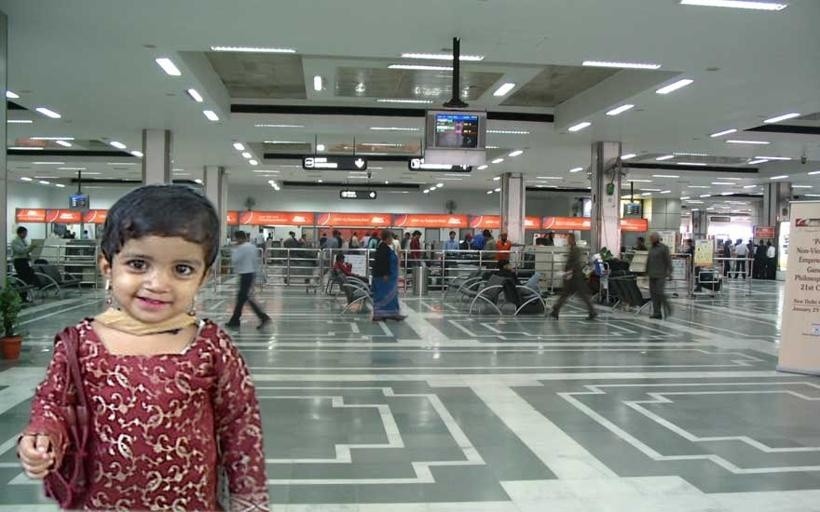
[{"xmin": 413, "ymin": 266, "xmax": 429, "ymax": 297}]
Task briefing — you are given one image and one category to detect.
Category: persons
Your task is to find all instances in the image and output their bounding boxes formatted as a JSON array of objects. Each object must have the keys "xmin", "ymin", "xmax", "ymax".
[
  {"xmin": 16, "ymin": 185, "xmax": 268, "ymax": 512},
  {"xmin": 257, "ymin": 229, "xmax": 273, "ymax": 266},
  {"xmin": 11, "ymin": 228, "xmax": 36, "ymax": 304},
  {"xmin": 63, "ymin": 229, "xmax": 91, "ymax": 256},
  {"xmin": 281, "ymin": 229, "xmax": 378, "ymax": 288},
  {"xmin": 224, "ymin": 230, "xmax": 272, "ymax": 335},
  {"xmin": 372, "ymin": 229, "xmax": 432, "ymax": 321},
  {"xmin": 446, "ymin": 230, "xmax": 701, "ymax": 319},
  {"xmin": 719, "ymin": 238, "xmax": 778, "ymax": 280}
]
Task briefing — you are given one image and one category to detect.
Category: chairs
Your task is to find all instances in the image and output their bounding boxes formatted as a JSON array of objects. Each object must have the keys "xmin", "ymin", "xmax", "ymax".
[{"xmin": 611, "ymin": 275, "xmax": 654, "ymax": 317}]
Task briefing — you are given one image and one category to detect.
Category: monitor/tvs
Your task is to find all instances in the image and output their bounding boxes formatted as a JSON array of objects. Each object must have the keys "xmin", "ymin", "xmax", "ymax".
[
  {"xmin": 70, "ymin": 193, "xmax": 89, "ymax": 212},
  {"xmin": 426, "ymin": 110, "xmax": 487, "ymax": 150}
]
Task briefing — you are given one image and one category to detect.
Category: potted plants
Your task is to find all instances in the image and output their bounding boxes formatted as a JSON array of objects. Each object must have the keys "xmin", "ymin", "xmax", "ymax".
[{"xmin": 0, "ymin": 285, "xmax": 24, "ymax": 361}]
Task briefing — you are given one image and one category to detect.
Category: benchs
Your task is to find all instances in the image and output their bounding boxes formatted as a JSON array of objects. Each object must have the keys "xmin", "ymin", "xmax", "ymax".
[
  {"xmin": 444, "ymin": 270, "xmax": 546, "ymax": 317},
  {"xmin": 8, "ymin": 258, "xmax": 82, "ymax": 301},
  {"xmin": 329, "ymin": 269, "xmax": 374, "ymax": 315}
]
